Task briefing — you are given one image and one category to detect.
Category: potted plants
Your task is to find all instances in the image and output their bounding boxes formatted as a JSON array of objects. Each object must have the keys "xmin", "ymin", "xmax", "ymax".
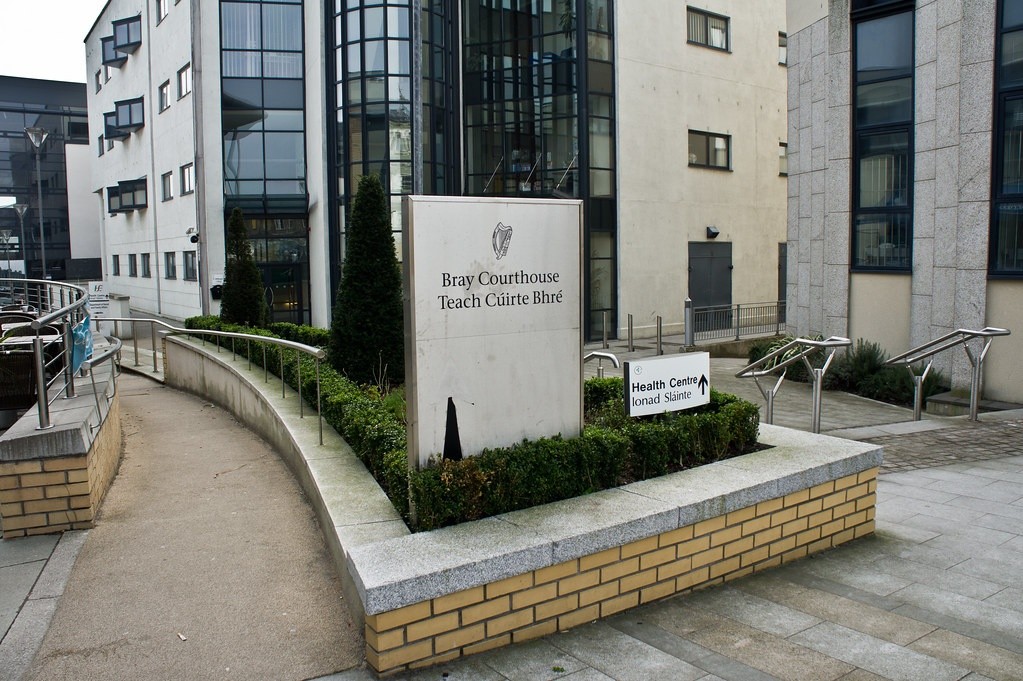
[{"xmin": 558, "ymin": 0, "xmax": 593, "ymax": 60}]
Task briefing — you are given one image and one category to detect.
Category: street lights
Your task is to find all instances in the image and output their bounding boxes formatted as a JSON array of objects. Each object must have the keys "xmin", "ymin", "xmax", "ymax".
[
  {"xmin": 1, "ymin": 229, "xmax": 13, "ymax": 278},
  {"xmin": 24, "ymin": 126, "xmax": 50, "ymax": 279},
  {"xmin": 12, "ymin": 203, "xmax": 30, "ymax": 279}
]
